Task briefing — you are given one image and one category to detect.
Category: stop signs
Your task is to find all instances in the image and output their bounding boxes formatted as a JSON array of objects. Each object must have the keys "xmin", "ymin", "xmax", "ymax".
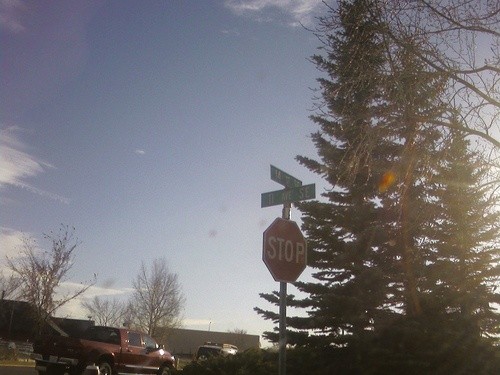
[{"xmin": 261, "ymin": 217, "xmax": 308, "ymax": 282}]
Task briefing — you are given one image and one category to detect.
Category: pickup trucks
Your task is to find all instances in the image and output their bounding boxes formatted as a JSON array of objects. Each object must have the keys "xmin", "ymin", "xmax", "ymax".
[{"xmin": 32, "ymin": 324, "xmax": 176, "ymax": 375}]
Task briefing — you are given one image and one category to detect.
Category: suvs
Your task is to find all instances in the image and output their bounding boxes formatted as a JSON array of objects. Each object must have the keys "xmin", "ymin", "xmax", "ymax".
[{"xmin": 193, "ymin": 340, "xmax": 239, "ymax": 365}]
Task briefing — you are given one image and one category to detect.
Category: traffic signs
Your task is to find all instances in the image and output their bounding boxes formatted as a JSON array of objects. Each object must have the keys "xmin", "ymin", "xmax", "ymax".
[
  {"xmin": 270, "ymin": 163, "xmax": 302, "ymax": 192},
  {"xmin": 259, "ymin": 182, "xmax": 317, "ymax": 210}
]
